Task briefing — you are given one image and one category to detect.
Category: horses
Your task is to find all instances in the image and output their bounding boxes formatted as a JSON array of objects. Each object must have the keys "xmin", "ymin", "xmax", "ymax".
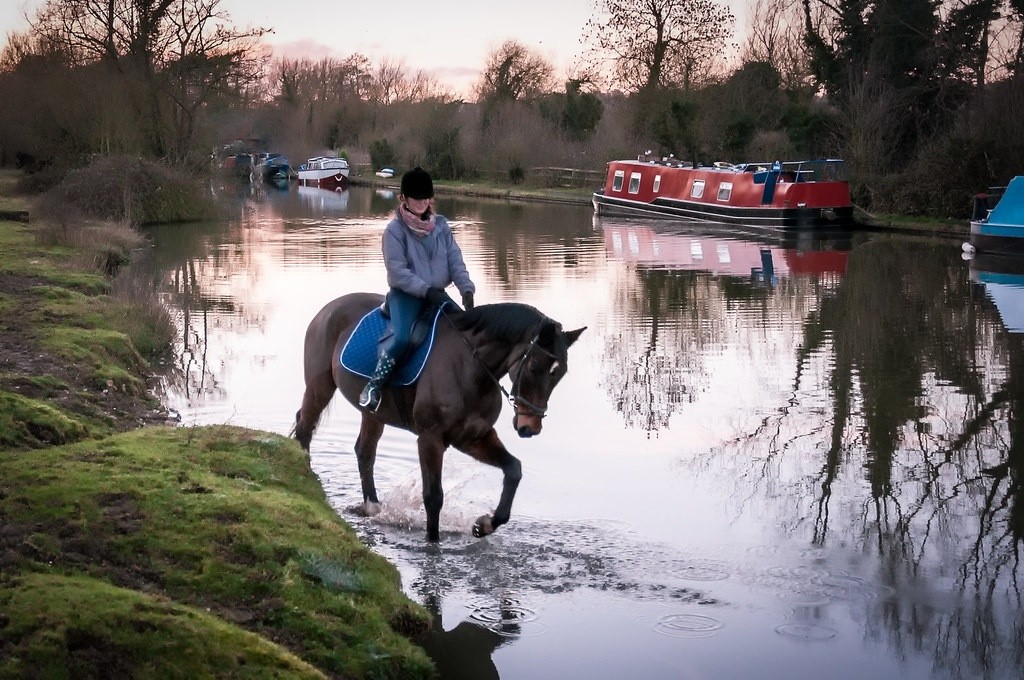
[{"xmin": 295, "ymin": 292, "xmax": 587, "ymax": 543}]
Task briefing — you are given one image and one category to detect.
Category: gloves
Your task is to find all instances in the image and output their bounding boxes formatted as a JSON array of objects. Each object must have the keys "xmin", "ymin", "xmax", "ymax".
[
  {"xmin": 461, "ymin": 291, "xmax": 474, "ymax": 312},
  {"xmin": 426, "ymin": 287, "xmax": 446, "ymax": 302}
]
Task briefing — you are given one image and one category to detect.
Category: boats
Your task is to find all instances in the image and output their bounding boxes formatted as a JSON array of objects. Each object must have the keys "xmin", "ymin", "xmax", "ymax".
[
  {"xmin": 297, "ymin": 156, "xmax": 351, "ymax": 186},
  {"xmin": 970, "ymin": 176, "xmax": 1024, "ymax": 256},
  {"xmin": 298, "ymin": 180, "xmax": 349, "ymax": 210},
  {"xmin": 968, "ymin": 253, "xmax": 1024, "ymax": 334},
  {"xmin": 591, "ymin": 149, "xmax": 854, "ymax": 228},
  {"xmin": 223, "ymin": 152, "xmax": 289, "ymax": 179},
  {"xmin": 592, "ymin": 212, "xmax": 855, "ymax": 279}
]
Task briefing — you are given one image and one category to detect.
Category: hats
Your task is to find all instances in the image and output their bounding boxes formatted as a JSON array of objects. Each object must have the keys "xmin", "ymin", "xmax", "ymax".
[{"xmin": 401, "ymin": 167, "xmax": 434, "ymax": 198}]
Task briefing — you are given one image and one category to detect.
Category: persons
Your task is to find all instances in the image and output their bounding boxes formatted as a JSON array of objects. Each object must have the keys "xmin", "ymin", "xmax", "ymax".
[{"xmin": 360, "ymin": 167, "xmax": 473, "ymax": 408}]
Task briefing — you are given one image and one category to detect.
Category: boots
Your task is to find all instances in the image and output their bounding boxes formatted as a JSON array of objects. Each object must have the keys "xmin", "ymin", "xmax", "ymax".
[{"xmin": 359, "ymin": 349, "xmax": 399, "ymax": 409}]
